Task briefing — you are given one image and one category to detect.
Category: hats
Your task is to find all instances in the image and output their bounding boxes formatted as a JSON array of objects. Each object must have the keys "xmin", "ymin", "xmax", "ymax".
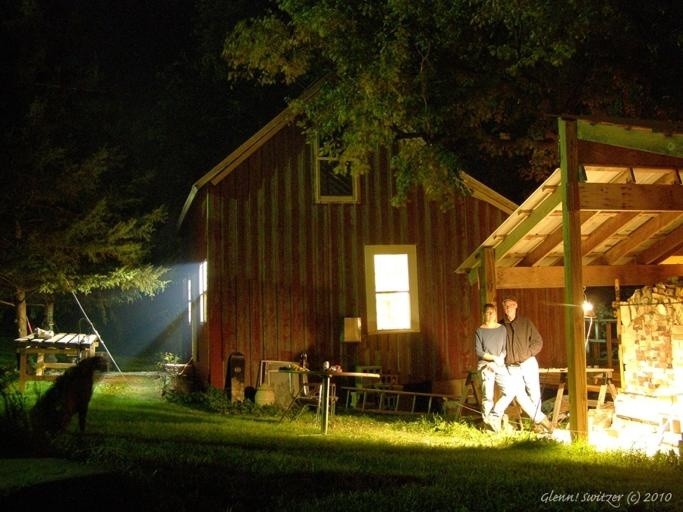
[{"xmin": 502, "ymin": 296, "xmax": 517, "ymax": 303}]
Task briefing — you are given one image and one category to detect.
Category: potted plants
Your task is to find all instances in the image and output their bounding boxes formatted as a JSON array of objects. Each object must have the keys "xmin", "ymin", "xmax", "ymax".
[{"xmin": 161, "ymin": 351, "xmax": 192, "ymax": 394}]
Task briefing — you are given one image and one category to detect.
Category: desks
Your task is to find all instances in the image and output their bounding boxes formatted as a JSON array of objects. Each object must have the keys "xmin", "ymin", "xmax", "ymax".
[
  {"xmin": 15, "ymin": 332, "xmax": 97, "ymax": 393},
  {"xmin": 279, "ymin": 369, "xmax": 380, "ymax": 435},
  {"xmin": 456, "ymin": 369, "xmax": 617, "ymax": 432}
]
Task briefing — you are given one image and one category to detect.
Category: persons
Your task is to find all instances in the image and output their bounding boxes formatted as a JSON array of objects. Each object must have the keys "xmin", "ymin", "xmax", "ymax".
[
  {"xmin": 498, "ymin": 297, "xmax": 563, "ymax": 440},
  {"xmin": 472, "ymin": 304, "xmax": 516, "ymax": 433}
]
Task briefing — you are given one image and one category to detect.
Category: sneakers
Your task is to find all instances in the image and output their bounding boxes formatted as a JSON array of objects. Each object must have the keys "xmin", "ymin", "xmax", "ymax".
[
  {"xmin": 534, "ymin": 416, "xmax": 554, "ymax": 434},
  {"xmin": 488, "ymin": 413, "xmax": 509, "ymax": 432}
]
